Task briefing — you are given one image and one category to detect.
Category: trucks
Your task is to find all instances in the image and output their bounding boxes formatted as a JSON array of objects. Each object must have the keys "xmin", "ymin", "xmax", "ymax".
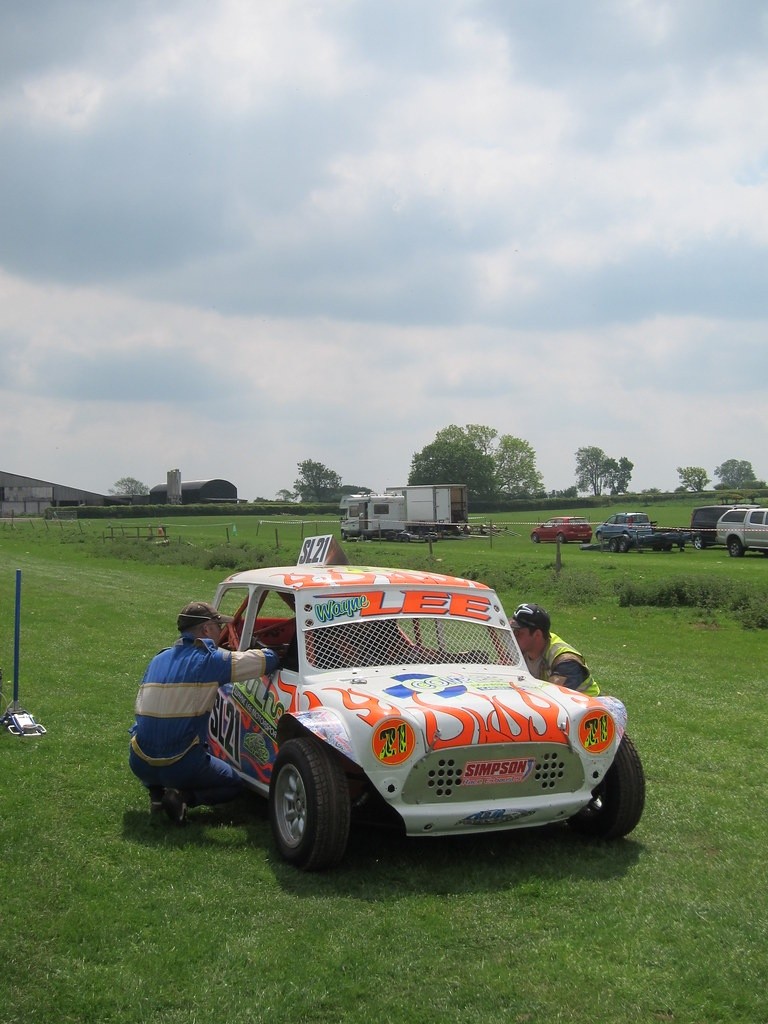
[{"xmin": 339, "ymin": 492, "xmax": 406, "ymax": 540}]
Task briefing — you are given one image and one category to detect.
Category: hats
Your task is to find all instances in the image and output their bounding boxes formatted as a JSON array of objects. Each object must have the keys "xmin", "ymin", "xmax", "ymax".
[
  {"xmin": 177, "ymin": 601, "xmax": 234, "ymax": 631},
  {"xmin": 510, "ymin": 604, "xmax": 550, "ymax": 629}
]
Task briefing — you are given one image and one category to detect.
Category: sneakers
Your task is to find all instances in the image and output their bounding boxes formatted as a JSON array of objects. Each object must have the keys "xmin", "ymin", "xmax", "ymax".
[
  {"xmin": 149, "ymin": 794, "xmax": 164, "ymax": 813},
  {"xmin": 162, "ymin": 787, "xmax": 192, "ymax": 828}
]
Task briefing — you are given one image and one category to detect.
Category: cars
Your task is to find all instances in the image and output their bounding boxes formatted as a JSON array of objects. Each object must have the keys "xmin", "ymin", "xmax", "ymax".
[
  {"xmin": 209, "ymin": 565, "xmax": 645, "ymax": 870},
  {"xmin": 530, "ymin": 517, "xmax": 593, "ymax": 544},
  {"xmin": 595, "ymin": 512, "xmax": 653, "ymax": 541}
]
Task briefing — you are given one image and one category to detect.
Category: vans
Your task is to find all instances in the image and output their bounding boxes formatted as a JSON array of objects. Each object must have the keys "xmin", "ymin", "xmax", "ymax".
[{"xmin": 690, "ymin": 504, "xmax": 760, "ymax": 550}]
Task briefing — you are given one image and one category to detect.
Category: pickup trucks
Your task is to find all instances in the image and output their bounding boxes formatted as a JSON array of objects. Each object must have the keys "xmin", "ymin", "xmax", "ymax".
[{"xmin": 715, "ymin": 508, "xmax": 768, "ymax": 557}]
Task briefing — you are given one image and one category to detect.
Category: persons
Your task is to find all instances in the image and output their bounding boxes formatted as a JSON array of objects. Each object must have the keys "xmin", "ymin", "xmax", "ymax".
[
  {"xmin": 129, "ymin": 602, "xmax": 280, "ymax": 826},
  {"xmin": 507, "ymin": 603, "xmax": 601, "ymax": 698}
]
